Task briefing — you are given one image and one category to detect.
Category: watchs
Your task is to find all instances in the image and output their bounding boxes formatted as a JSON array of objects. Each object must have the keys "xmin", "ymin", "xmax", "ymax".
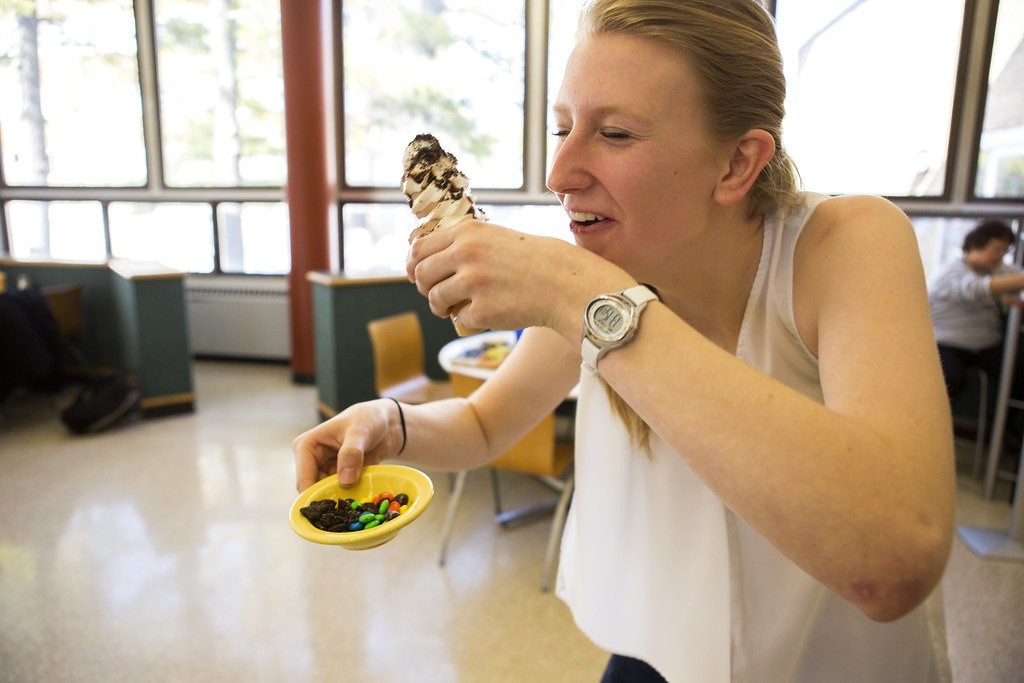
[{"xmin": 580, "ymin": 285, "xmax": 659, "ymax": 376}]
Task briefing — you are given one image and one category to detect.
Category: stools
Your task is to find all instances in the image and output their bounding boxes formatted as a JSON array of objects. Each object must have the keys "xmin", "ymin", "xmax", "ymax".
[{"xmin": 946, "ymin": 364, "xmax": 989, "ymax": 480}]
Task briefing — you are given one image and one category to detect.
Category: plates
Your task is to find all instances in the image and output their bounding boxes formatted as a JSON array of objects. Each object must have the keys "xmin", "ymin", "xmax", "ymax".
[{"xmin": 289, "ymin": 464, "xmax": 434, "ymax": 551}]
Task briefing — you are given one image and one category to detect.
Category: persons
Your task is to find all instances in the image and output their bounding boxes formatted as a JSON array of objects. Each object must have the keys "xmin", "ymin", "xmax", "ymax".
[
  {"xmin": 291, "ymin": 1, "xmax": 957, "ymax": 683},
  {"xmin": 927, "ymin": 220, "xmax": 1024, "ymax": 416}
]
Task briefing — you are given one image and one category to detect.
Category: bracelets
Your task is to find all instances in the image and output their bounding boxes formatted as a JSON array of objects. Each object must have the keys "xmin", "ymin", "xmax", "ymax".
[{"xmin": 389, "ymin": 397, "xmax": 408, "ymax": 456}]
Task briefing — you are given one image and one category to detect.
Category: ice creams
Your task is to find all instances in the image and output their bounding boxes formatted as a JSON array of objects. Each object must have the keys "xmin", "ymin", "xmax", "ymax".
[{"xmin": 400, "ymin": 132, "xmax": 496, "ymax": 337}]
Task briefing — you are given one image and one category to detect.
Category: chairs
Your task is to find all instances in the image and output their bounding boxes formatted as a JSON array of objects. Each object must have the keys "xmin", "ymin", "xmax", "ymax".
[
  {"xmin": 437, "ymin": 370, "xmax": 575, "ymax": 591},
  {"xmin": 367, "ymin": 310, "xmax": 459, "ymax": 493}
]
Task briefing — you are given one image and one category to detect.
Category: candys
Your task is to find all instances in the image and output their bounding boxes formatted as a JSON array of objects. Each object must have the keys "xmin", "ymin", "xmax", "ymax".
[{"xmin": 298, "ymin": 492, "xmax": 409, "ymax": 531}]
